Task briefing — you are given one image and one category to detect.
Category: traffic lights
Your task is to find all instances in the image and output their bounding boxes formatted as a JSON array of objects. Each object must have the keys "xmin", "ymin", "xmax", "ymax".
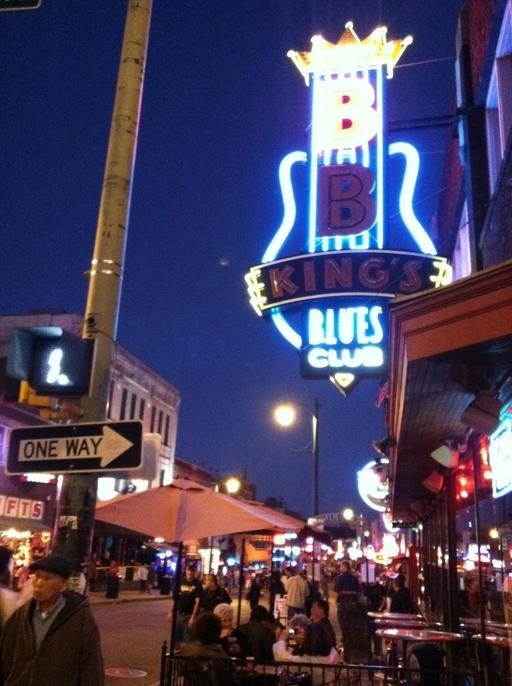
[{"xmin": 8, "ymin": 325, "xmax": 97, "ymax": 398}]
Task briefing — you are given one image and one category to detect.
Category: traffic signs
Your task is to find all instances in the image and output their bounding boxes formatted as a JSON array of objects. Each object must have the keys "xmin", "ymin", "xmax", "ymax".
[{"xmin": 5, "ymin": 420, "xmax": 143, "ymax": 477}]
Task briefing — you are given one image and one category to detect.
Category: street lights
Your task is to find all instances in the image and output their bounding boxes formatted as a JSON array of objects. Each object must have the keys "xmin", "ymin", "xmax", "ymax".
[
  {"xmin": 208, "ymin": 477, "xmax": 240, "ymax": 575},
  {"xmin": 273, "ymin": 397, "xmax": 319, "ymax": 593},
  {"xmin": 363, "ymin": 530, "xmax": 371, "ymax": 587},
  {"xmin": 2, "ymin": 526, "xmax": 31, "ymax": 590}
]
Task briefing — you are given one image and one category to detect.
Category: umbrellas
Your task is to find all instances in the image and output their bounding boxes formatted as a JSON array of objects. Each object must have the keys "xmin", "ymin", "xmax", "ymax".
[{"xmin": 93, "ymin": 475, "xmax": 333, "ymax": 658}]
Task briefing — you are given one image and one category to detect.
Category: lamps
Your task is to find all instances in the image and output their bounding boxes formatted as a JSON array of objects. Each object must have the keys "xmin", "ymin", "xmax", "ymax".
[
  {"xmin": 423, "ymin": 469, "xmax": 445, "ymax": 493},
  {"xmin": 430, "ymin": 434, "xmax": 462, "ymax": 469},
  {"xmin": 374, "ymin": 435, "xmax": 398, "ymax": 454},
  {"xmin": 456, "ymin": 379, "xmax": 507, "ymax": 436}
]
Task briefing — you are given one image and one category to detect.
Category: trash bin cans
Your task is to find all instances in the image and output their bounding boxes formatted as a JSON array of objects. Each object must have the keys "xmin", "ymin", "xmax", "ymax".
[
  {"xmin": 105, "ymin": 575, "xmax": 119, "ymax": 599},
  {"xmin": 159, "ymin": 577, "xmax": 171, "ymax": 595}
]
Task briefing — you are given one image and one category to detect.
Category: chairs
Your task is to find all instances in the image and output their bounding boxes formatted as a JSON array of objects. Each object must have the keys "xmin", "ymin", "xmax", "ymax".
[{"xmin": 166, "ymin": 594, "xmax": 467, "ymax": 686}]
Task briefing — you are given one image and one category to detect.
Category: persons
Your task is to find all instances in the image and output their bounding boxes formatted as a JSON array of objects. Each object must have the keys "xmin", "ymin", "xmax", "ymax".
[
  {"xmin": 456, "ymin": 563, "xmax": 511, "ymax": 628},
  {"xmin": 0, "ymin": 555, "xmax": 105, "ymax": 686},
  {"xmin": 107, "ymin": 551, "xmax": 420, "ymax": 685}
]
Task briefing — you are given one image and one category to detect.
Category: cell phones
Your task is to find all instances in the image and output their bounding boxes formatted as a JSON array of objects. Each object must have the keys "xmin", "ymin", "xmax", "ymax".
[{"xmin": 288, "ymin": 627, "xmax": 297, "ymax": 645}]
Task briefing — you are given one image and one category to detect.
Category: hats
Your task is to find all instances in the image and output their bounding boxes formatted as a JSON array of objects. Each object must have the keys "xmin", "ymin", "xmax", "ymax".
[
  {"xmin": 213, "ymin": 603, "xmax": 234, "ymax": 618},
  {"xmin": 28, "ymin": 556, "xmax": 73, "ymax": 579},
  {"xmin": 186, "ymin": 562, "xmax": 196, "ymax": 569}
]
